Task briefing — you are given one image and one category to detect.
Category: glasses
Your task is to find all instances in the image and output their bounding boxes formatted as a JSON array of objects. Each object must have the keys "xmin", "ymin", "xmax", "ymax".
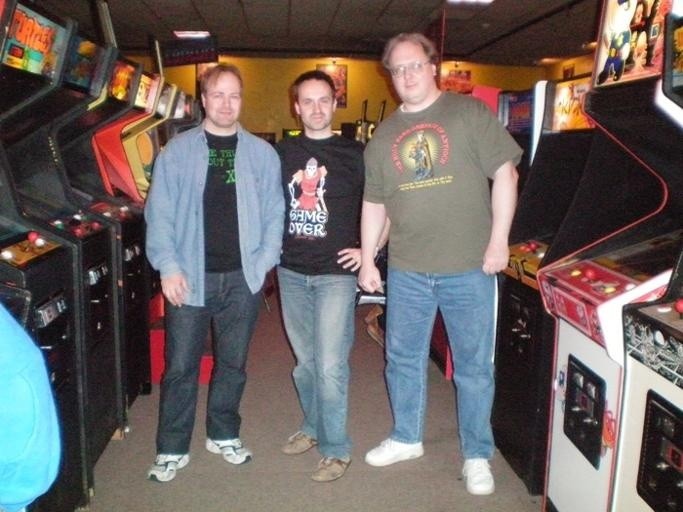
[{"xmin": 388, "ymin": 56, "xmax": 433, "ymax": 80}]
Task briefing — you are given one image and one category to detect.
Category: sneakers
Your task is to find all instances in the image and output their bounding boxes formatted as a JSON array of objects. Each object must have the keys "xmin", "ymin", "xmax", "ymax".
[
  {"xmin": 281, "ymin": 431, "xmax": 318, "ymax": 455},
  {"xmin": 310, "ymin": 454, "xmax": 351, "ymax": 482},
  {"xmin": 205, "ymin": 437, "xmax": 252, "ymax": 464},
  {"xmin": 147, "ymin": 453, "xmax": 189, "ymax": 482},
  {"xmin": 365, "ymin": 438, "xmax": 424, "ymax": 467},
  {"xmin": 463, "ymin": 458, "xmax": 495, "ymax": 495}
]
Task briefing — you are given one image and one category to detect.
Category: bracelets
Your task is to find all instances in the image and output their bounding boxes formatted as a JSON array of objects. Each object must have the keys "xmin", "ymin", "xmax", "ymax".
[{"xmin": 374, "ymin": 245, "xmax": 382, "ymax": 253}]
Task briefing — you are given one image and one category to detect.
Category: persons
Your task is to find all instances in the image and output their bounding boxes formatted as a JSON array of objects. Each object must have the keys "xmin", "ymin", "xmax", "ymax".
[
  {"xmin": 353, "ymin": 32, "xmax": 528, "ymax": 496},
  {"xmin": 271, "ymin": 69, "xmax": 392, "ymax": 486},
  {"xmin": 139, "ymin": 61, "xmax": 285, "ymax": 483}
]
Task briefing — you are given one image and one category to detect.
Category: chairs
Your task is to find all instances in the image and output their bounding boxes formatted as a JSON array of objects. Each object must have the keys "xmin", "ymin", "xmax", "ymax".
[
  {"xmin": 263, "ymin": 275, "xmax": 276, "ymax": 312},
  {"xmin": 355, "ymin": 255, "xmax": 388, "ymax": 304}
]
